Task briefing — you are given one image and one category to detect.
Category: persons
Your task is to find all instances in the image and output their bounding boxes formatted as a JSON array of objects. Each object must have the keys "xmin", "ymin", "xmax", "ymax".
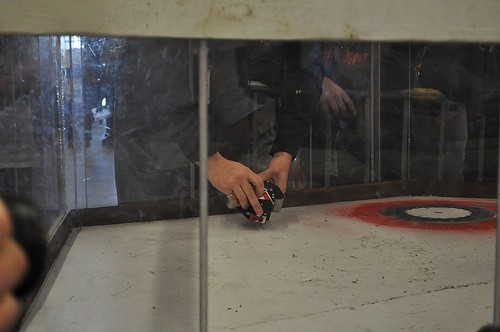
[
  {"xmin": 114, "ymin": 38, "xmax": 326, "ymax": 216},
  {"xmin": 317, "ymin": 42, "xmax": 499, "ymax": 183}
]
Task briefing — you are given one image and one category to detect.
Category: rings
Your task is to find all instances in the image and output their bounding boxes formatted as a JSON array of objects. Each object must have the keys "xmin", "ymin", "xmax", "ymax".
[{"xmin": 227, "ymin": 193, "xmax": 233, "ymax": 198}]
